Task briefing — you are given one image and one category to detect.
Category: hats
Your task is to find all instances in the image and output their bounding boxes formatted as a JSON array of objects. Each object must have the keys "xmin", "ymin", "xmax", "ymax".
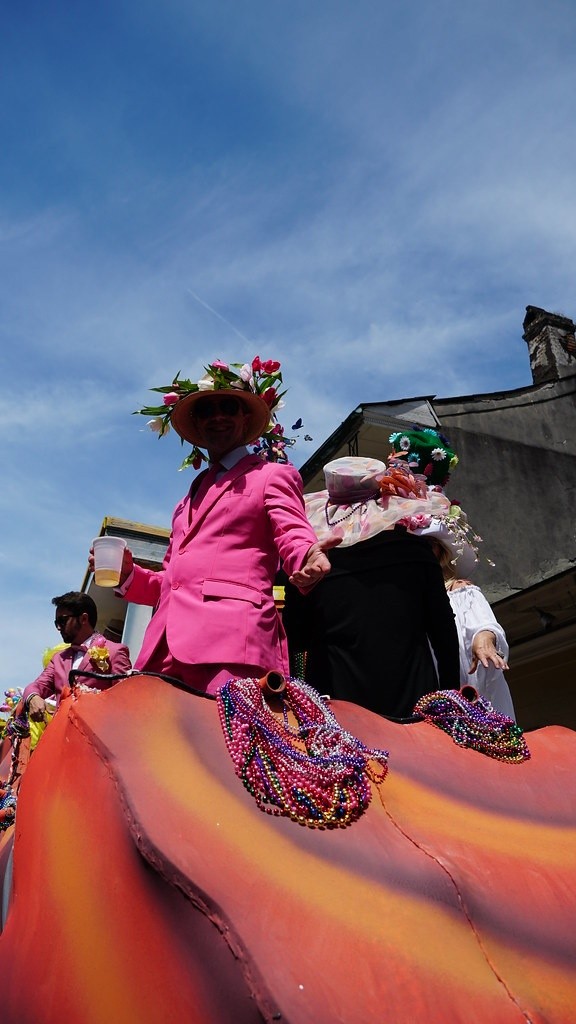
[
  {"xmin": 131, "ymin": 355, "xmax": 313, "ymax": 471},
  {"xmin": 302, "ymin": 456, "xmax": 451, "ymax": 548}
]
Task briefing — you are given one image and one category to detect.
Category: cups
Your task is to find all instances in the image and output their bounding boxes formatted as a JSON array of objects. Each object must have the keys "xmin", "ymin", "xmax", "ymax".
[{"xmin": 92, "ymin": 536, "xmax": 128, "ymax": 587}]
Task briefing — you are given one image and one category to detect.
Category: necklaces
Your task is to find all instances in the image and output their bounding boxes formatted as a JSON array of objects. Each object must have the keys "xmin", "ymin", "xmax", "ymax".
[
  {"xmin": 216, "ymin": 676, "xmax": 389, "ymax": 827},
  {"xmin": 415, "ymin": 688, "xmax": 530, "ymax": 763}
]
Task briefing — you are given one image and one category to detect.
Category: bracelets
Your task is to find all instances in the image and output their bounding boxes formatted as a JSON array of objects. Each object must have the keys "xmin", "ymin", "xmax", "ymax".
[{"xmin": 28, "ymin": 694, "xmax": 37, "ymax": 704}]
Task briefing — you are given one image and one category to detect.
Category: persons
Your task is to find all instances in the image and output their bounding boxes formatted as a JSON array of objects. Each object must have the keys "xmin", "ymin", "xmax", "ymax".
[
  {"xmin": 22, "ymin": 592, "xmax": 131, "ymax": 723},
  {"xmin": 281, "ymin": 496, "xmax": 460, "ymax": 717},
  {"xmin": 86, "ymin": 394, "xmax": 341, "ymax": 694},
  {"xmin": 422, "ymin": 536, "xmax": 519, "ymax": 727}
]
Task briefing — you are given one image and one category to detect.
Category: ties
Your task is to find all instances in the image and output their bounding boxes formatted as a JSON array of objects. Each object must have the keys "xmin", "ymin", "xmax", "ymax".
[{"xmin": 189, "ymin": 464, "xmax": 223, "ymax": 526}]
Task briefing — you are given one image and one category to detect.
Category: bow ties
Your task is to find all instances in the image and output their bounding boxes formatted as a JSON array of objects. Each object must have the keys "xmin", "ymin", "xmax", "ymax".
[{"xmin": 60, "ymin": 645, "xmax": 88, "ymax": 661}]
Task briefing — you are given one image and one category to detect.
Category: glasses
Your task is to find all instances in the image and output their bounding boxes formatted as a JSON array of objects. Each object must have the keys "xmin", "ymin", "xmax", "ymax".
[{"xmin": 54, "ymin": 614, "xmax": 79, "ymax": 628}]
